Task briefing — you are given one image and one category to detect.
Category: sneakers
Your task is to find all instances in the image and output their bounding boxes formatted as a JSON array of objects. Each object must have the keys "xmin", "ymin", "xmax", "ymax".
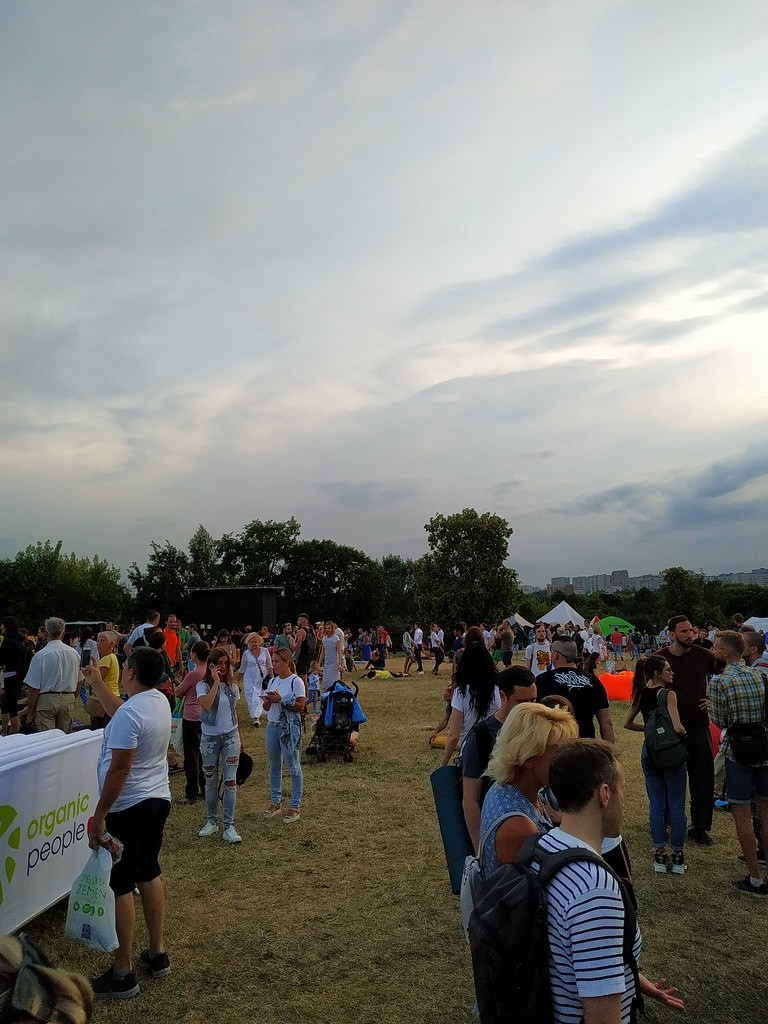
[
  {"xmin": 653, "ymin": 848, "xmax": 687, "ymax": 874},
  {"xmin": 223, "ymin": 825, "xmax": 242, "ymax": 843},
  {"xmin": 730, "ymin": 851, "xmax": 768, "ymax": 899},
  {"xmin": 90, "ymin": 950, "xmax": 172, "ymax": 998},
  {"xmin": 199, "ymin": 820, "xmax": 219, "ymax": 836}
]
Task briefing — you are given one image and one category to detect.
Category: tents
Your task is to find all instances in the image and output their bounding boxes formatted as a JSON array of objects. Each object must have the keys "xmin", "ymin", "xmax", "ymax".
[
  {"xmin": 743, "ymin": 617, "xmax": 768, "ymax": 651},
  {"xmin": 502, "ymin": 600, "xmax": 635, "ymax": 637}
]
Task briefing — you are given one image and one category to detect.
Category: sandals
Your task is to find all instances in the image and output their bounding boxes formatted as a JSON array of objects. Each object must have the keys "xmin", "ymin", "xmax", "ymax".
[{"xmin": 263, "ymin": 803, "xmax": 301, "ymax": 823}]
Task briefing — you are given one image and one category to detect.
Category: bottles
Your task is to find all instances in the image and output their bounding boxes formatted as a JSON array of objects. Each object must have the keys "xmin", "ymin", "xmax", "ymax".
[{"xmin": 103, "ymin": 831, "xmax": 123, "ymax": 865}]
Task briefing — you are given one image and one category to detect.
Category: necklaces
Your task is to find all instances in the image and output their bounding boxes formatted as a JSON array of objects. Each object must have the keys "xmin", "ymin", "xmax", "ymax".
[{"xmin": 516, "ymin": 783, "xmax": 544, "ymax": 816}]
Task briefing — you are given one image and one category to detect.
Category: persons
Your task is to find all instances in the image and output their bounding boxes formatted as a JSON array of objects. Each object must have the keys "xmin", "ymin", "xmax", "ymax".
[
  {"xmin": 623, "ymin": 616, "xmax": 768, "ymax": 898},
  {"xmin": 403, "ymin": 613, "xmax": 755, "ymax": 678},
  {"xmin": 0, "ymin": 610, "xmax": 393, "ymax": 842},
  {"xmin": 428, "ymin": 627, "xmax": 685, "ymax": 1024},
  {"xmin": 88, "ymin": 647, "xmax": 172, "ymax": 998}
]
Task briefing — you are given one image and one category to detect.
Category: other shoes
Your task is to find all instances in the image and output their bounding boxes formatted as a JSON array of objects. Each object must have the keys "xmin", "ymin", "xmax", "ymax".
[
  {"xmin": 253, "ymin": 720, "xmax": 260, "ymax": 728},
  {"xmin": 168, "ymin": 761, "xmax": 185, "ymax": 774},
  {"xmin": 175, "ymin": 792, "xmax": 206, "ymax": 805},
  {"xmin": 403, "ymin": 669, "xmax": 442, "ymax": 676},
  {"xmin": 314, "ymin": 710, "xmax": 321, "ymax": 714},
  {"xmin": 688, "ymin": 827, "xmax": 716, "ymax": 846}
]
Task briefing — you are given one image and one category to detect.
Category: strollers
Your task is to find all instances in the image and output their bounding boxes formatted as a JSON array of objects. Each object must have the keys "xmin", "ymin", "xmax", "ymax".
[{"xmin": 311, "ymin": 679, "xmax": 358, "ymax": 765}]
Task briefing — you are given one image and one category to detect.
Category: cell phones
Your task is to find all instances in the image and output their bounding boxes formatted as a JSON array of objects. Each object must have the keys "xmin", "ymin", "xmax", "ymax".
[
  {"xmin": 80, "ymin": 647, "xmax": 92, "ymax": 669},
  {"xmin": 266, "ymin": 691, "xmax": 275, "ymax": 695},
  {"xmin": 208, "ymin": 662, "xmax": 216, "ymax": 669}
]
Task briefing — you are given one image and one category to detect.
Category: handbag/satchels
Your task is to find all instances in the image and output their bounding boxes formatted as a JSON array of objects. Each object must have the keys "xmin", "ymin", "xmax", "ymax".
[
  {"xmin": 170, "ymin": 699, "xmax": 183, "ymax": 749},
  {"xmin": 84, "ymin": 696, "xmax": 105, "ymax": 717},
  {"xmin": 605, "ymin": 661, "xmax": 615, "ymax": 674},
  {"xmin": 231, "ymin": 644, "xmax": 240, "ymax": 662},
  {"xmin": 64, "ymin": 845, "xmax": 119, "ymax": 952},
  {"xmin": 262, "ymin": 674, "xmax": 272, "ymax": 690},
  {"xmin": 729, "ymin": 721, "xmax": 768, "ymax": 765}
]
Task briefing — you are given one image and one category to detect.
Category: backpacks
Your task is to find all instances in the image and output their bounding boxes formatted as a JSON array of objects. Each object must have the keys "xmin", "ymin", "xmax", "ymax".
[
  {"xmin": 644, "ymin": 689, "xmax": 689, "ymax": 772},
  {"xmin": 272, "ymin": 676, "xmax": 309, "ymax": 734},
  {"xmin": 460, "ymin": 812, "xmax": 637, "ymax": 1024},
  {"xmin": 132, "ymin": 627, "xmax": 146, "ymax": 648},
  {"xmin": 301, "ymin": 625, "xmax": 317, "ymax": 654},
  {"xmin": 218, "ymin": 752, "xmax": 253, "ymax": 804}
]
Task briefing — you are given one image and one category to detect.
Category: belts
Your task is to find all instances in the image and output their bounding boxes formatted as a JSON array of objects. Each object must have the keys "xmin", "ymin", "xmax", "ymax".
[{"xmin": 39, "ymin": 691, "xmax": 74, "ymax": 695}]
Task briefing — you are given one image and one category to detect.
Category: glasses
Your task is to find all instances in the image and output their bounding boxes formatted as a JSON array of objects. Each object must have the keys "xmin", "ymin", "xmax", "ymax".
[
  {"xmin": 555, "ymin": 650, "xmax": 582, "ymax": 664},
  {"xmin": 662, "ymin": 667, "xmax": 671, "ymax": 673},
  {"xmin": 711, "ymin": 646, "xmax": 726, "ymax": 653}
]
